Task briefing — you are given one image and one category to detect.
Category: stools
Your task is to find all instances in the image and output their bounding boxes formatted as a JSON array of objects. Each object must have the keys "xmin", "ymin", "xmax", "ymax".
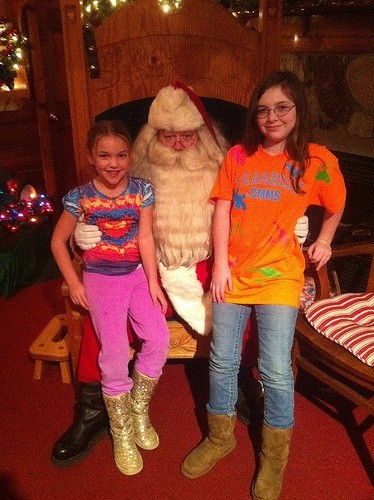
[{"xmin": 29, "ymin": 313, "xmax": 72, "ymax": 383}]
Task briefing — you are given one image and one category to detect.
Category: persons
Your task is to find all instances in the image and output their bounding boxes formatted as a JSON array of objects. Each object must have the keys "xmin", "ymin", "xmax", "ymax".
[
  {"xmin": 51, "ymin": 82, "xmax": 309, "ymax": 466},
  {"xmin": 52, "ymin": 120, "xmax": 171, "ymax": 475},
  {"xmin": 179, "ymin": 70, "xmax": 347, "ymax": 500}
]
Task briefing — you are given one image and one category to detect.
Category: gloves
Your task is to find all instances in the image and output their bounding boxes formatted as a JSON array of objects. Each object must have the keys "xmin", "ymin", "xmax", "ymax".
[
  {"xmin": 74, "ymin": 213, "xmax": 102, "ymax": 250},
  {"xmin": 294, "ymin": 216, "xmax": 309, "ymax": 243}
]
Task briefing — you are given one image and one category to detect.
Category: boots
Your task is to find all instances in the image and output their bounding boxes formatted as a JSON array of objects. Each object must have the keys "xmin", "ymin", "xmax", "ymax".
[
  {"xmin": 181, "ymin": 410, "xmax": 237, "ymax": 480},
  {"xmin": 101, "ymin": 390, "xmax": 144, "ymax": 475},
  {"xmin": 235, "ymin": 364, "xmax": 266, "ymax": 425},
  {"xmin": 251, "ymin": 422, "xmax": 294, "ymax": 500},
  {"xmin": 128, "ymin": 366, "xmax": 162, "ymax": 450},
  {"xmin": 50, "ymin": 381, "xmax": 110, "ymax": 467}
]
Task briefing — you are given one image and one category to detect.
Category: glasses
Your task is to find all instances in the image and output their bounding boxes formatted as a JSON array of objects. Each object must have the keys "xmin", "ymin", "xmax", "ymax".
[
  {"xmin": 164, "ymin": 134, "xmax": 196, "ymax": 148},
  {"xmin": 255, "ymin": 104, "xmax": 297, "ymax": 119}
]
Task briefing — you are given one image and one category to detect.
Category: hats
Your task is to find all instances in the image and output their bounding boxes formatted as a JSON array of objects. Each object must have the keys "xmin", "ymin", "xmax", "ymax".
[{"xmin": 130, "ymin": 81, "xmax": 228, "ymax": 165}]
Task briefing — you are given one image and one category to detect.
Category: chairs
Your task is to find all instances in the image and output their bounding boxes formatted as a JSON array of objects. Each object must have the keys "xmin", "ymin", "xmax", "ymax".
[{"xmin": 60, "ymin": 92, "xmax": 374, "ymax": 415}]
